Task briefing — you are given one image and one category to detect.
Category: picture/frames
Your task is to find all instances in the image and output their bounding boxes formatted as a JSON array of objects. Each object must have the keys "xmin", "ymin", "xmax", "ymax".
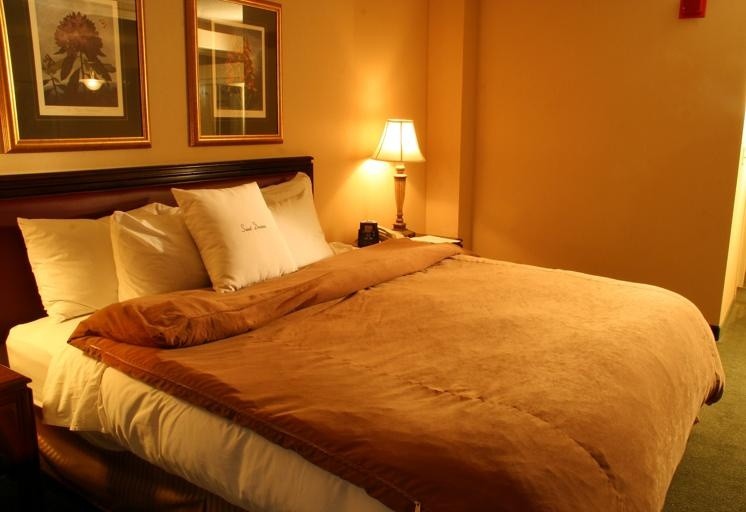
[
  {"xmin": 184, "ymin": 0, "xmax": 284, "ymax": 146},
  {"xmin": 0, "ymin": 0, "xmax": 152, "ymax": 154},
  {"xmin": 24, "ymin": 1, "xmax": 130, "ymax": 122},
  {"xmin": 210, "ymin": 19, "xmax": 267, "ymax": 119}
]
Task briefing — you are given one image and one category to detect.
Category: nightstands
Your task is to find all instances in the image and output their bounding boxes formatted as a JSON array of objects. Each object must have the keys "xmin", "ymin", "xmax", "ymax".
[
  {"xmin": 349, "ymin": 231, "xmax": 463, "ymax": 249},
  {"xmin": 0, "ymin": 364, "xmax": 41, "ymax": 512}
]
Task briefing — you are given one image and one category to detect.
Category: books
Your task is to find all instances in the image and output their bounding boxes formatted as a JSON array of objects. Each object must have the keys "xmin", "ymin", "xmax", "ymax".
[{"xmin": 410, "ymin": 234, "xmax": 462, "ymax": 245}]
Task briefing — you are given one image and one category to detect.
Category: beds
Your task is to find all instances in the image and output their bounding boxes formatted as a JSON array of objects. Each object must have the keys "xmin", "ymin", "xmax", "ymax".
[{"xmin": 0, "ymin": 155, "xmax": 727, "ymax": 512}]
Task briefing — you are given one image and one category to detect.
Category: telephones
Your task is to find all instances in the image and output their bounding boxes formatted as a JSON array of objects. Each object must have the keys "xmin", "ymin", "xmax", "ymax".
[{"xmin": 378, "ymin": 225, "xmax": 403, "ymax": 240}]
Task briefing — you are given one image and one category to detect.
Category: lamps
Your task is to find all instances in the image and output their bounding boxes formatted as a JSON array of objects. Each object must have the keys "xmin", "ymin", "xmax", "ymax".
[
  {"xmin": 78, "ymin": 61, "xmax": 106, "ymax": 92},
  {"xmin": 370, "ymin": 118, "xmax": 425, "ymax": 239}
]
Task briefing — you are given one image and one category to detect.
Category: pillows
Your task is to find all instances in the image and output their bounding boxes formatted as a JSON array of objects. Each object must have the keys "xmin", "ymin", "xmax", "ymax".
[
  {"xmin": 260, "ymin": 172, "xmax": 334, "ymax": 270},
  {"xmin": 170, "ymin": 183, "xmax": 298, "ymax": 295},
  {"xmin": 16, "ymin": 215, "xmax": 122, "ymax": 324},
  {"xmin": 109, "ymin": 205, "xmax": 210, "ymax": 304}
]
